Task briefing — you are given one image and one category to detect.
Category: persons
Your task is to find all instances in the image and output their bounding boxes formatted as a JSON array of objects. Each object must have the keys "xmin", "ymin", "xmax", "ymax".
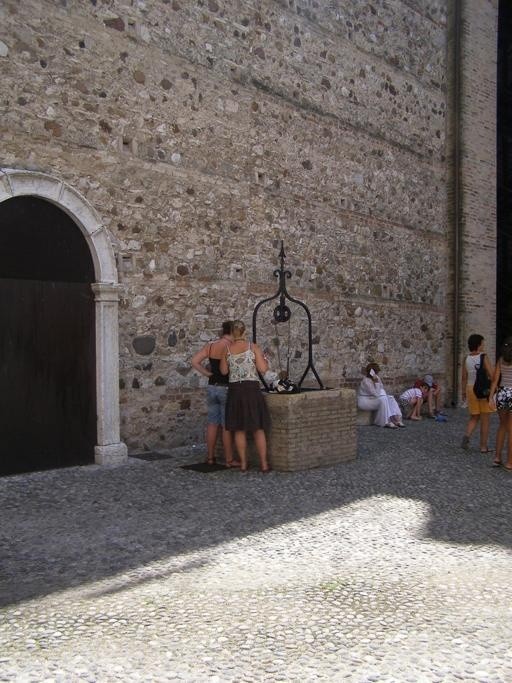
[
  {"xmin": 414, "ymin": 373, "xmax": 449, "ymax": 419},
  {"xmin": 219, "ymin": 319, "xmax": 272, "ymax": 471},
  {"xmin": 190, "ymin": 320, "xmax": 241, "ymax": 468},
  {"xmin": 357, "ymin": 362, "xmax": 406, "ymax": 429},
  {"xmin": 397, "ymin": 381, "xmax": 430, "ymax": 421},
  {"xmin": 488, "ymin": 337, "xmax": 512, "ymax": 472},
  {"xmin": 460, "ymin": 333, "xmax": 496, "ymax": 452}
]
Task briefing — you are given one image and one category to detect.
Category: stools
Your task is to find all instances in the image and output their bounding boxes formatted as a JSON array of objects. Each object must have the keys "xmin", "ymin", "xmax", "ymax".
[{"xmin": 355, "ymin": 407, "xmax": 375, "ymax": 427}]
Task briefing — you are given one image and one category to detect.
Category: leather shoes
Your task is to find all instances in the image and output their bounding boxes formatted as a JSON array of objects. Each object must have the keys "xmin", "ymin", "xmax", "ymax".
[{"xmin": 384, "ymin": 420, "xmax": 406, "ymax": 429}]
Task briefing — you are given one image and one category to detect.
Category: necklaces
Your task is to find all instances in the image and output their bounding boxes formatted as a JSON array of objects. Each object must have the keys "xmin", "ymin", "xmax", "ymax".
[
  {"xmin": 234, "ymin": 338, "xmax": 246, "ymax": 343},
  {"xmin": 220, "ymin": 336, "xmax": 232, "ymax": 344}
]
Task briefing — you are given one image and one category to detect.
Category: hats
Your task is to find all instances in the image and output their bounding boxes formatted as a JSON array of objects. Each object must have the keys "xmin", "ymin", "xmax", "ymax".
[{"xmin": 423, "ymin": 375, "xmax": 434, "ymax": 387}]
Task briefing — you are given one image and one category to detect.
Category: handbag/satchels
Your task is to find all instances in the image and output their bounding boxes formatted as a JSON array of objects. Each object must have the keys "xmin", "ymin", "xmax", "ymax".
[{"xmin": 475, "ymin": 368, "xmax": 497, "ymax": 398}]
